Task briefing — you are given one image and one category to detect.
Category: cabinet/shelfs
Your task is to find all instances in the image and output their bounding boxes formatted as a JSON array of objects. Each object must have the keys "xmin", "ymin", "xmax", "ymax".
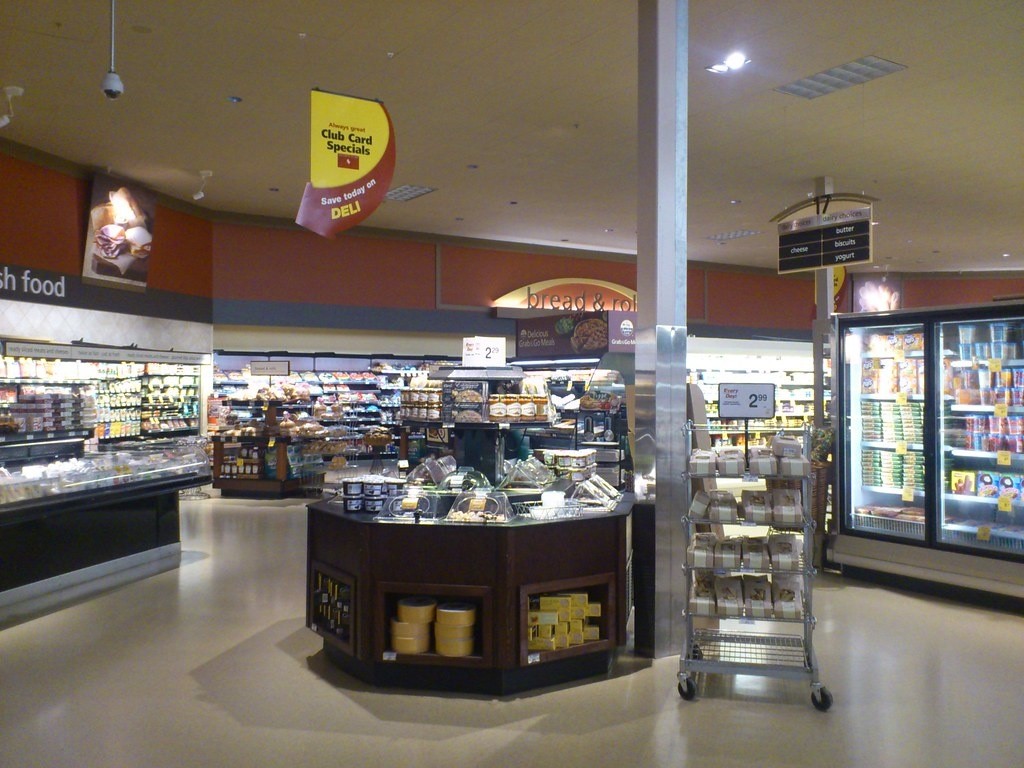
[
  {"xmin": 139, "ymin": 373, "xmax": 200, "ymax": 438},
  {"xmin": 213, "ymin": 381, "xmax": 401, "ymax": 461},
  {"xmin": 522, "ymin": 380, "xmax": 612, "ymax": 439},
  {"xmin": 0, "ymin": 378, "xmax": 101, "ymax": 409},
  {"xmin": 687, "ymin": 367, "xmax": 831, "ymax": 434},
  {"xmin": 375, "ymin": 370, "xmax": 442, "ymax": 420}
]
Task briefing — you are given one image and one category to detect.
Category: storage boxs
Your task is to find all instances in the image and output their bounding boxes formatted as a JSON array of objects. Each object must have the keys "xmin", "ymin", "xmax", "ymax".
[
  {"xmin": 568, "ymin": 633, "xmax": 584, "ymax": 644},
  {"xmin": 687, "ymin": 488, "xmax": 805, "ymax": 619},
  {"xmin": 585, "ymin": 602, "xmax": 601, "ymax": 617},
  {"xmin": 584, "ymin": 626, "xmax": 599, "ymax": 640},
  {"xmin": 540, "ymin": 595, "xmax": 571, "ymax": 610},
  {"xmin": 571, "ymin": 606, "xmax": 585, "ymax": 620},
  {"xmin": 779, "ymin": 454, "xmax": 810, "ymax": 476},
  {"xmin": 688, "ymin": 450, "xmax": 717, "ymax": 475},
  {"xmin": 770, "ymin": 435, "xmax": 801, "ymax": 458},
  {"xmin": 528, "ymin": 634, "xmax": 556, "ymax": 650},
  {"xmin": 558, "ymin": 592, "xmax": 588, "ymax": 607},
  {"xmin": 748, "ymin": 447, "xmax": 778, "ymax": 476},
  {"xmin": 9, "ymin": 393, "xmax": 99, "ymax": 431},
  {"xmin": 555, "ymin": 634, "xmax": 569, "ymax": 648},
  {"xmin": 528, "ymin": 609, "xmax": 559, "ymax": 625},
  {"xmin": 538, "ymin": 625, "xmax": 554, "ymax": 639},
  {"xmin": 555, "ymin": 622, "xmax": 570, "ymax": 635},
  {"xmin": 569, "ymin": 618, "xmax": 588, "ymax": 633},
  {"xmin": 558, "ymin": 608, "xmax": 571, "ymax": 622},
  {"xmin": 717, "ymin": 449, "xmax": 747, "ymax": 475},
  {"xmin": 951, "ymin": 469, "xmax": 1024, "ymax": 501}
]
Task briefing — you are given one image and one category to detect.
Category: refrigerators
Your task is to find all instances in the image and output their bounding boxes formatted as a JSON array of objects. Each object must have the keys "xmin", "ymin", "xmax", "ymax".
[{"xmin": 826, "ymin": 299, "xmax": 1024, "ymax": 599}]
